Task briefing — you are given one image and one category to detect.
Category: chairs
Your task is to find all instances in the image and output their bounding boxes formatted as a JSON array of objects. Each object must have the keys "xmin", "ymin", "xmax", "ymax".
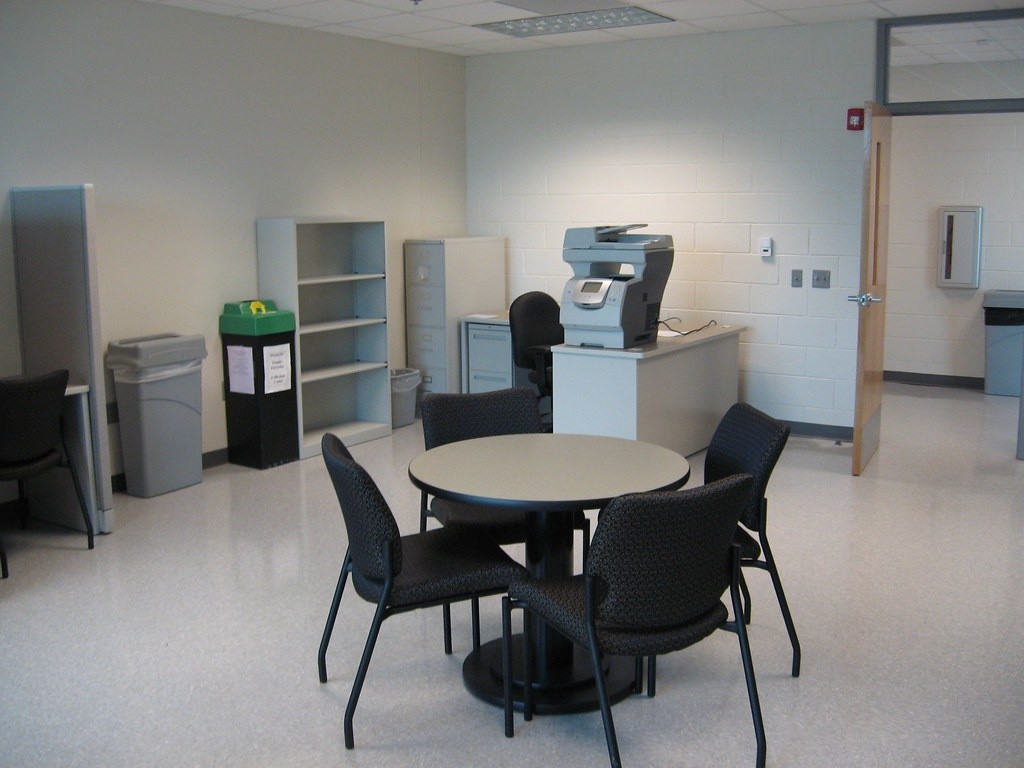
[
  {"xmin": 419, "ymin": 389, "xmax": 590, "ymax": 575},
  {"xmin": 502, "ymin": 471, "xmax": 767, "ymax": 768},
  {"xmin": 508, "ymin": 292, "xmax": 565, "ymax": 430},
  {"xmin": 597, "ymin": 402, "xmax": 801, "ymax": 677},
  {"xmin": 0, "ymin": 366, "xmax": 93, "ymax": 579},
  {"xmin": 317, "ymin": 433, "xmax": 525, "ymax": 750}
]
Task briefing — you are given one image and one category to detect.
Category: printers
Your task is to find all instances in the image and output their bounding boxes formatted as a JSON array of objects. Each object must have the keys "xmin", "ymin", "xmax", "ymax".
[{"xmin": 559, "ymin": 224, "xmax": 674, "ymax": 348}]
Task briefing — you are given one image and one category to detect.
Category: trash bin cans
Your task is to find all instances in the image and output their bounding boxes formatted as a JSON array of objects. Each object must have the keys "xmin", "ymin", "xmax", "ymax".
[
  {"xmin": 107, "ymin": 332, "xmax": 208, "ymax": 498},
  {"xmin": 982, "ymin": 291, "xmax": 1024, "ymax": 397},
  {"xmin": 391, "ymin": 367, "xmax": 423, "ymax": 427}
]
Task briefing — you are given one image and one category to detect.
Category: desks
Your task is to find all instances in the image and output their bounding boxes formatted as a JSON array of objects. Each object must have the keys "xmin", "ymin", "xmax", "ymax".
[
  {"xmin": 408, "ymin": 437, "xmax": 691, "ymax": 716},
  {"xmin": 550, "ymin": 321, "xmax": 747, "ymax": 458},
  {"xmin": 30, "ymin": 385, "xmax": 100, "ymax": 535}
]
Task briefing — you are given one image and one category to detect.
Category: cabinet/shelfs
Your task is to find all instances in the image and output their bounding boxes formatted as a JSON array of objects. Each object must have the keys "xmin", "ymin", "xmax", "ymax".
[
  {"xmin": 257, "ymin": 215, "xmax": 392, "ymax": 458},
  {"xmin": 459, "ymin": 316, "xmax": 537, "ymax": 396},
  {"xmin": 404, "ymin": 237, "xmax": 508, "ymax": 418}
]
